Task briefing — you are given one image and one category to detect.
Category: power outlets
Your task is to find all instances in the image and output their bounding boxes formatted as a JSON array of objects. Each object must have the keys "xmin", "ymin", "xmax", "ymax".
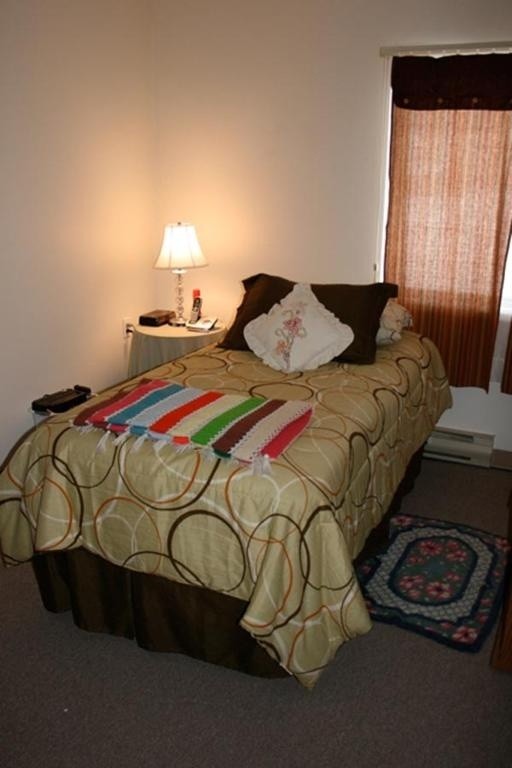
[{"xmin": 122, "ymin": 318, "xmax": 131, "ymax": 338}]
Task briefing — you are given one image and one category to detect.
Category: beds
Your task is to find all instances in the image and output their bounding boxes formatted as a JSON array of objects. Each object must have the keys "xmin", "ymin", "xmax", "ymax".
[{"xmin": 24, "ymin": 325, "xmax": 450, "ymax": 679}]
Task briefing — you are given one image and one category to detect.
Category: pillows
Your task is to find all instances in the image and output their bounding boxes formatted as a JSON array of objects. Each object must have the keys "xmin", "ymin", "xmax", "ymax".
[{"xmin": 213, "ymin": 273, "xmax": 414, "ymax": 376}]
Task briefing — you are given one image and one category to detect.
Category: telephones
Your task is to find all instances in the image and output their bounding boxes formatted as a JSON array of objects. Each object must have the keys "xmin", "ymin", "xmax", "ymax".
[{"xmin": 185, "ymin": 296, "xmax": 218, "ymax": 331}]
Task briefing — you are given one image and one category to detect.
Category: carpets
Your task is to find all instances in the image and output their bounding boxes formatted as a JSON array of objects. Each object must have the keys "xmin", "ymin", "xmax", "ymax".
[{"xmin": 352, "ymin": 510, "xmax": 512, "ymax": 656}]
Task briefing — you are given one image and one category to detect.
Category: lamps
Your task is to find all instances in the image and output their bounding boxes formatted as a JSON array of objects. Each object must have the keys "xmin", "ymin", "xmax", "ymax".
[{"xmin": 155, "ymin": 220, "xmax": 209, "ymax": 326}]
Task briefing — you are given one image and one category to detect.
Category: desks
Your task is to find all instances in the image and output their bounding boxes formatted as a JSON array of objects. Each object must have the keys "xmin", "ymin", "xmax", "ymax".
[{"xmin": 128, "ymin": 322, "xmax": 227, "ymax": 376}]
